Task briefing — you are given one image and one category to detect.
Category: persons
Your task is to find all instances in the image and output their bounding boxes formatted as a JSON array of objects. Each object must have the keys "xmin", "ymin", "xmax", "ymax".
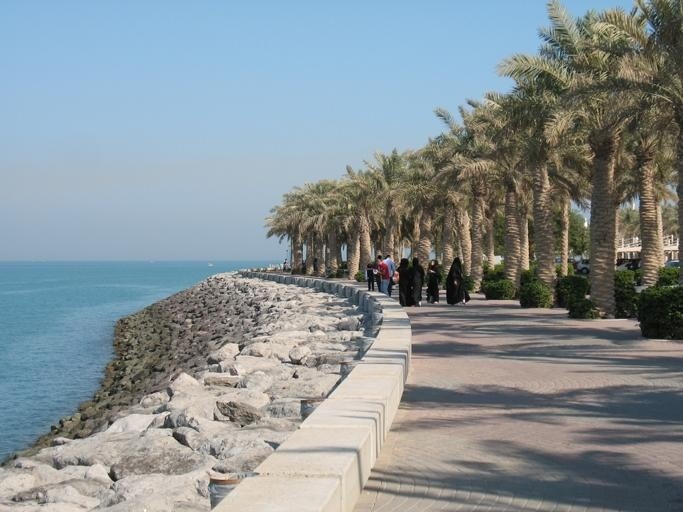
[{"xmin": 365, "ymin": 255, "xmax": 472, "ymax": 306}]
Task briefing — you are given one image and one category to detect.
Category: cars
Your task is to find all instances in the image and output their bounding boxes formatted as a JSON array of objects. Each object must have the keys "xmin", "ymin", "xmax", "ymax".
[{"xmin": 552, "ymin": 255, "xmax": 681, "ymax": 284}]
